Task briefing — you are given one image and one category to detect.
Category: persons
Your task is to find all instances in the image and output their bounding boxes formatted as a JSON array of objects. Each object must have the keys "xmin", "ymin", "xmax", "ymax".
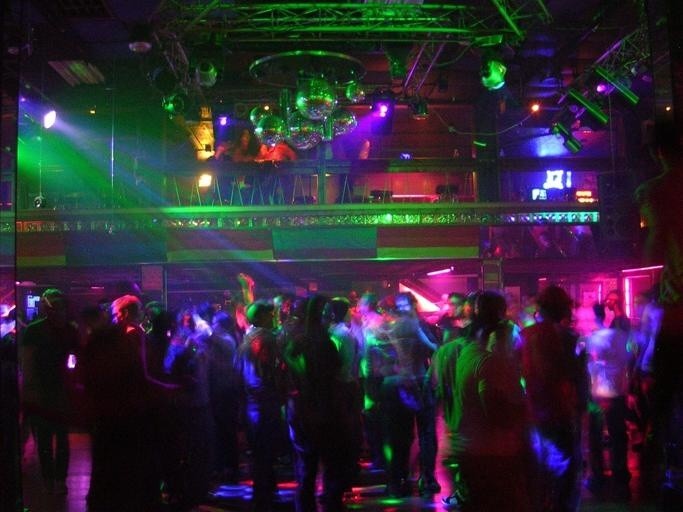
[
  {"xmin": 636, "ymin": 116, "xmax": 682, "ymax": 502},
  {"xmin": 213, "ymin": 128, "xmax": 298, "ymax": 203},
  {"xmin": 452, "ymin": 146, "xmax": 464, "ymax": 159},
  {"xmin": 497, "ymin": 135, "xmax": 515, "ymax": 177}
]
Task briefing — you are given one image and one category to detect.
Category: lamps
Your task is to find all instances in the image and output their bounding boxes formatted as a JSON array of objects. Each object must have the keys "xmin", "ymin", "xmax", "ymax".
[
  {"xmin": 128, "ymin": 21, "xmax": 154, "ymax": 55},
  {"xmin": 156, "ymin": 71, "xmax": 190, "ymax": 121},
  {"xmin": 552, "ymin": 16, "xmax": 652, "ymax": 154},
  {"xmin": 186, "ymin": 49, "xmax": 451, "ymax": 150},
  {"xmin": 479, "ymin": 35, "xmax": 507, "ymax": 92}
]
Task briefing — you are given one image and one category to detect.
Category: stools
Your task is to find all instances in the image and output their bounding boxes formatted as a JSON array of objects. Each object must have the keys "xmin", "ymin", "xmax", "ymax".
[
  {"xmin": 369, "ymin": 189, "xmax": 393, "ymax": 202},
  {"xmin": 435, "ymin": 184, "xmax": 460, "ymax": 203}
]
